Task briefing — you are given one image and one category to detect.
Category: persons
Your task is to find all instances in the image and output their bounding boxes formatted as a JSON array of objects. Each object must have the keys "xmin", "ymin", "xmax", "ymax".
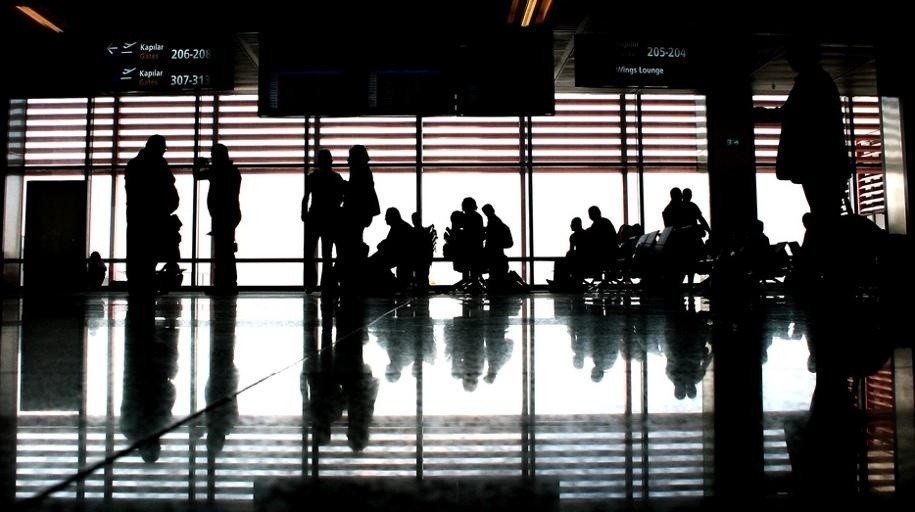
[
  {"xmin": 749, "ymin": 41, "xmax": 851, "ymax": 265},
  {"xmin": 802, "ymin": 213, "xmax": 811, "ymax": 229},
  {"xmin": 193, "ymin": 143, "xmax": 241, "ymax": 296},
  {"xmin": 125, "ymin": 134, "xmax": 180, "ymax": 317}
]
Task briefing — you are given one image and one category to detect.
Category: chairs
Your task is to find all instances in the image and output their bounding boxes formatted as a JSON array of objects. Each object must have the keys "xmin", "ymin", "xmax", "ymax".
[{"xmin": 581, "ymin": 226, "xmax": 807, "ymax": 308}]
